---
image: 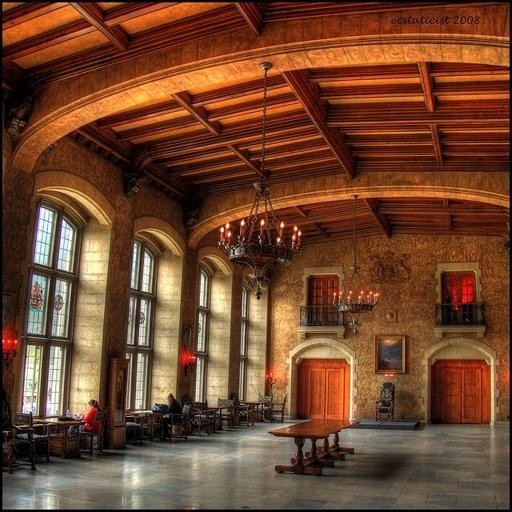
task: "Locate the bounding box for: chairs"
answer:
[1,392,287,474]
[375,381,394,421]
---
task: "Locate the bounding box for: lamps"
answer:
[264,371,278,387]
[0,325,21,365]
[184,350,198,376]
[330,191,379,335]
[214,60,306,300]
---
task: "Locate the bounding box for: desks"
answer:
[268,417,361,476]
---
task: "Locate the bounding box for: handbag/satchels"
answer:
[152,404,168,413]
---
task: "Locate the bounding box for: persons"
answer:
[66,398,102,439]
[158,393,183,438]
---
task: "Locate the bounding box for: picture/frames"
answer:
[375,335,405,374]
